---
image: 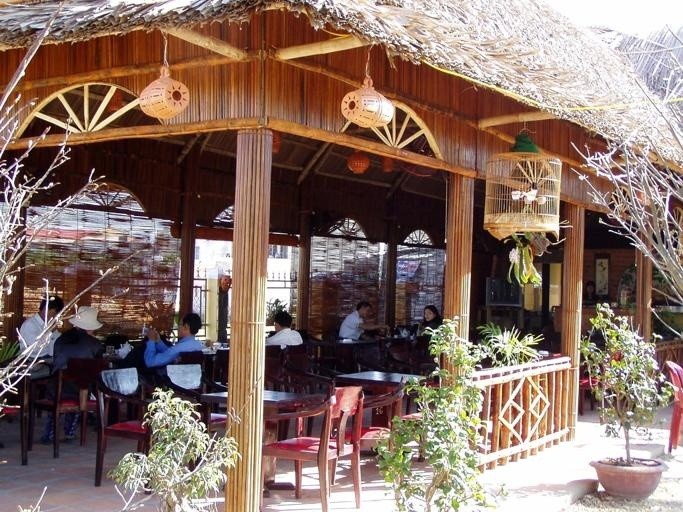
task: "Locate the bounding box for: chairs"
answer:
[665,360,683,453]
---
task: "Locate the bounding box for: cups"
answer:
[106,346,114,356]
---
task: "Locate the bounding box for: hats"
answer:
[68,306,103,331]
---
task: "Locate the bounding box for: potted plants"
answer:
[578,300,683,502]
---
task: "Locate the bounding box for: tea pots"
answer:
[120,341,133,351]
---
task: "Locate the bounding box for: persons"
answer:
[265,311,303,350]
[581,281,601,305]
[128,312,201,387]
[37,305,104,443]
[421,304,442,343]
[18,296,63,371]
[337,301,390,371]
